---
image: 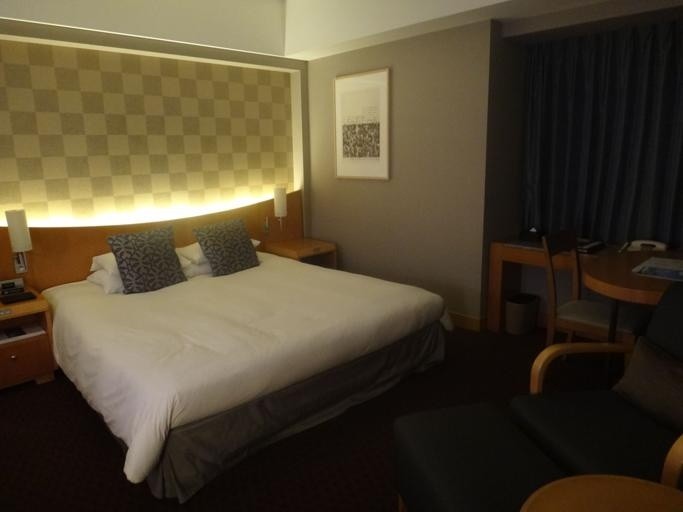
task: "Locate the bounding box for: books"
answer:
[632,257,683,281]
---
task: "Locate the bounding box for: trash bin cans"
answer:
[504,293,539,334]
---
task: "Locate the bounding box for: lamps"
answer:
[273,186,288,235]
[5,207,32,275]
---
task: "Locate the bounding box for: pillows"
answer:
[87,216,261,296]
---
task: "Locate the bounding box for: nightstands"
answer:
[262,239,338,270]
[0,286,58,391]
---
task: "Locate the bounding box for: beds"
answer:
[37,237,456,511]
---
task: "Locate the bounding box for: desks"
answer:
[486,236,602,334]
[581,248,683,341]
[519,473,683,512]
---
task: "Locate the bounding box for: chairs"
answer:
[542,236,650,377]
[384,282,683,510]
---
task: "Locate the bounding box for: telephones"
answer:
[627,239,667,252]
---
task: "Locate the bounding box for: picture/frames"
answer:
[331,66,391,181]
